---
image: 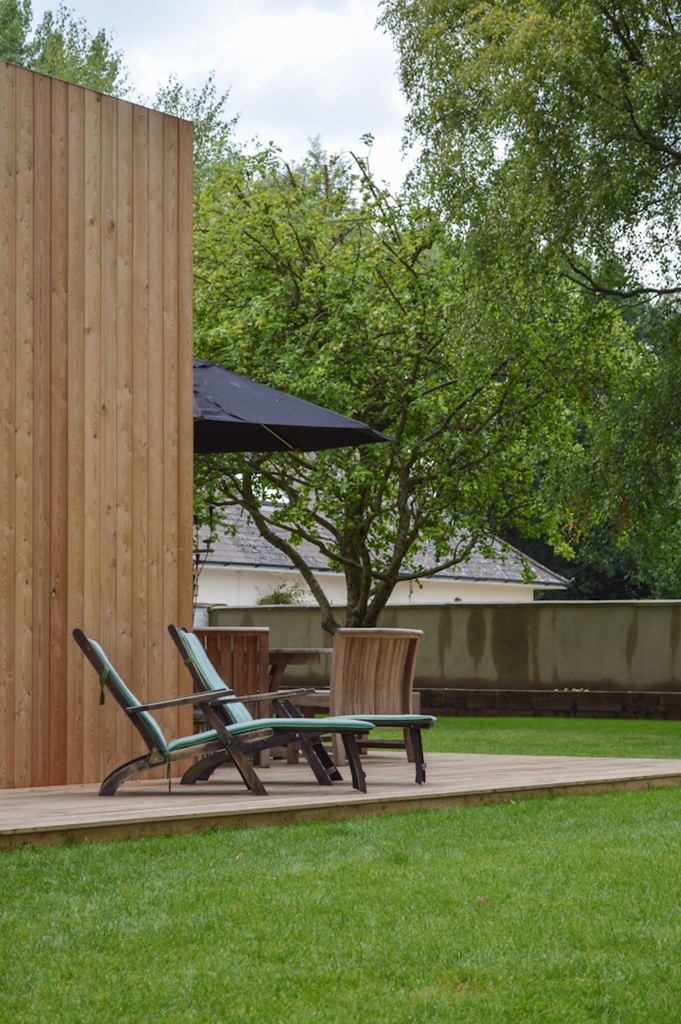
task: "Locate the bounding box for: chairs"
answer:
[72,627,375,793]
[193,627,272,767]
[168,623,437,785]
[286,627,424,766]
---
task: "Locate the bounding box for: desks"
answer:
[200,647,333,761]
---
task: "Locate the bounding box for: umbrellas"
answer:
[193,357,397,455]
